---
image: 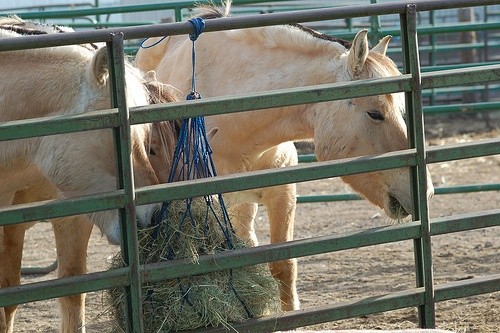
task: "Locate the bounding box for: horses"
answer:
[1,15,218,332]
[133,16,434,315]
